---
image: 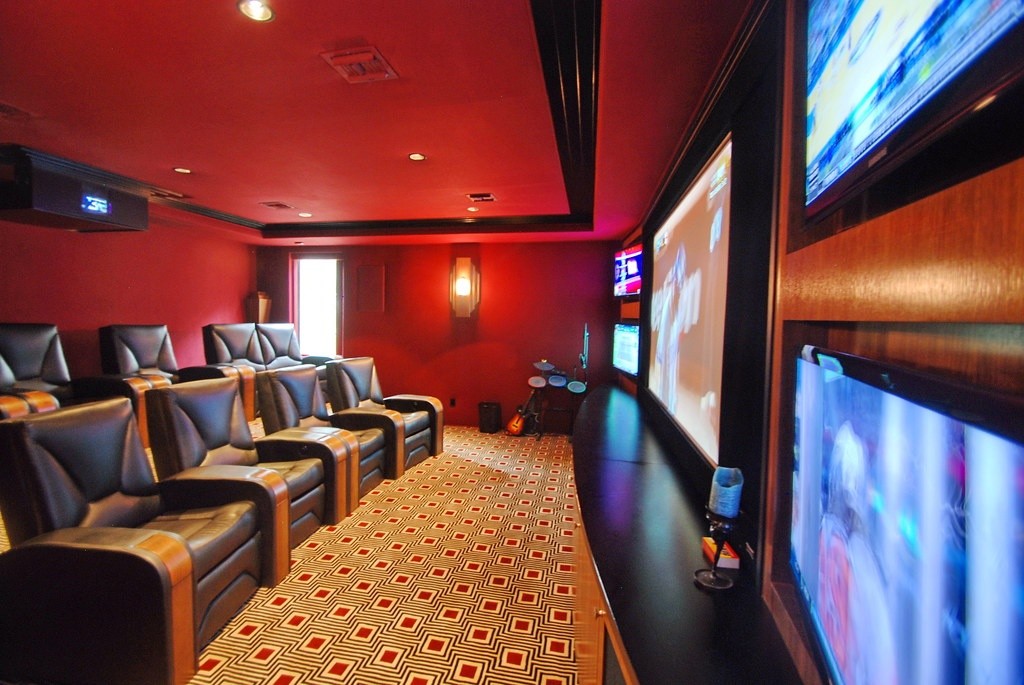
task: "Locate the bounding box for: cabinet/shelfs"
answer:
[569,378,800,685]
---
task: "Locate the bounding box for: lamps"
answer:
[448,256,480,319]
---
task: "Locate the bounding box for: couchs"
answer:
[0,320,444,685]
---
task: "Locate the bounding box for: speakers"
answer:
[478,402,501,433]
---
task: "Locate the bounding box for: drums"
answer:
[567,381,586,393]
[548,375,567,387]
[527,376,546,387]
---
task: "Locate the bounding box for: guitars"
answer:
[524,389,541,435]
[505,389,536,436]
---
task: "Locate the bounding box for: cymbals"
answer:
[533,362,555,371]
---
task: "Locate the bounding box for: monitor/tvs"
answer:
[611,113,761,501]
[788,345,1024,685]
[800,0,1024,228]
